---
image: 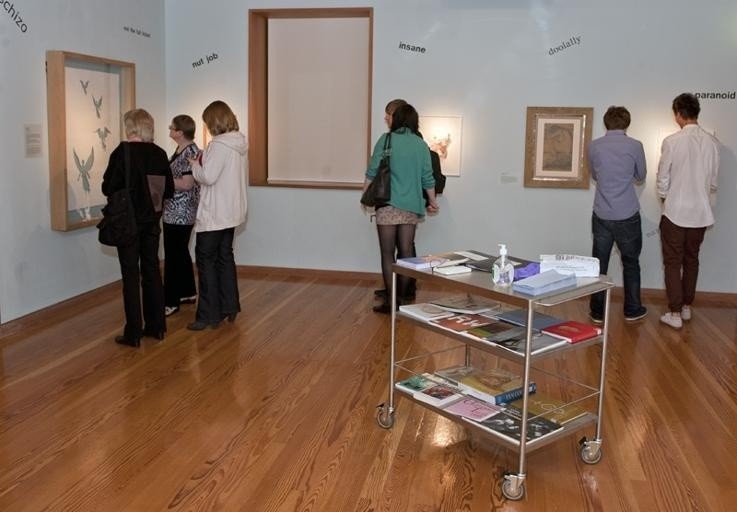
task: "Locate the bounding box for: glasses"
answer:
[169,126,175,130]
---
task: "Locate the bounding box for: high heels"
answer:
[219,311,237,322]
[187,320,219,330]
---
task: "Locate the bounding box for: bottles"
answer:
[491,243,514,288]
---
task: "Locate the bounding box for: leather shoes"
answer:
[375,290,386,296]
[142,328,164,340]
[373,303,399,313]
[115,336,141,347]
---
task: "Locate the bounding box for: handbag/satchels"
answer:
[96,188,137,246]
[361,133,391,206]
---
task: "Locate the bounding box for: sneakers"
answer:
[624,307,648,320]
[588,312,603,323]
[180,295,197,304]
[681,305,691,320]
[660,312,682,329]
[165,306,180,316]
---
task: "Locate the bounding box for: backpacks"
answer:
[422,147,445,208]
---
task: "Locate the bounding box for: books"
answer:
[391,364,588,445]
[399,292,602,356]
[395,249,577,296]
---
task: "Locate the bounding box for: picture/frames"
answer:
[418,113,464,178]
[524,106,593,189]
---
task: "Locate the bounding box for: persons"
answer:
[101,108,176,348]
[586,104,650,325]
[656,92,722,329]
[362,103,441,315]
[187,99,249,332]
[384,99,447,302]
[162,114,203,318]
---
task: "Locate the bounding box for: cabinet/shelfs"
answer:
[375,249,617,501]
